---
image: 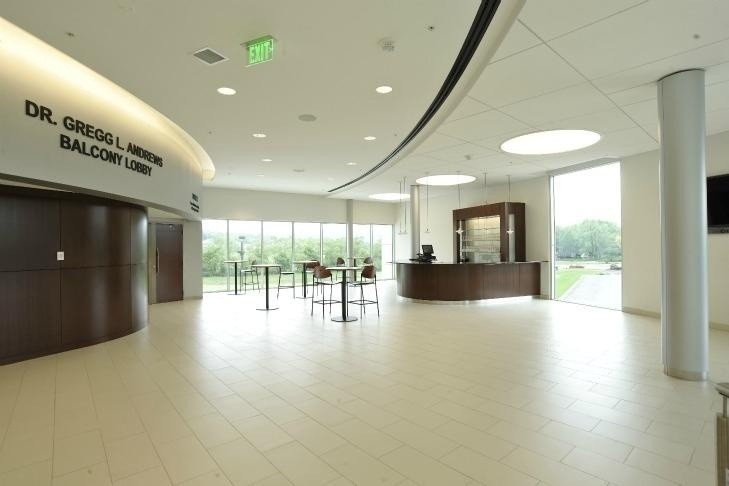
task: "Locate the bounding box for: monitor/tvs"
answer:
[707,174,729,234]
[422,245,433,255]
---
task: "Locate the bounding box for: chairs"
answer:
[224,258,381,324]
